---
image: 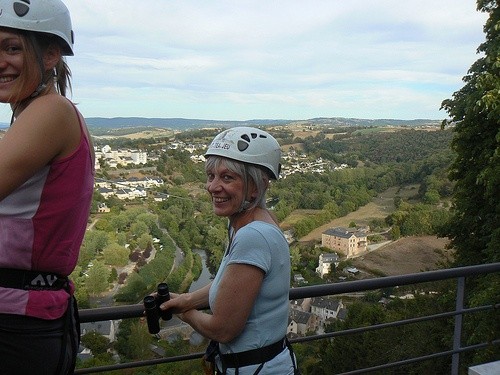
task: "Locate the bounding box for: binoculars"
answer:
[143,283,172,334]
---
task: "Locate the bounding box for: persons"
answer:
[0,0,94,375]
[146,127,298,375]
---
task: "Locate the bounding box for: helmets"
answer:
[0,0,74,56]
[203,127,281,181]
